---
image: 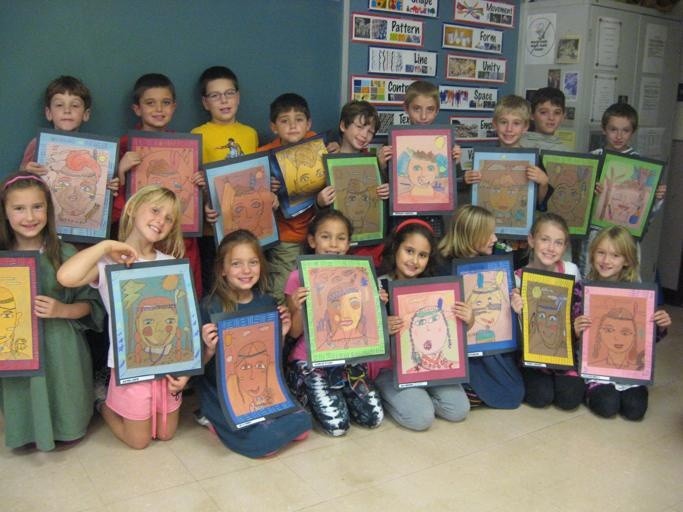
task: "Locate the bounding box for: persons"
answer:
[0,67,671,460]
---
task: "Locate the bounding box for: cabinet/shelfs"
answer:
[514,0,683,283]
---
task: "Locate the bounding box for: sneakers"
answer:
[191,407,208,428]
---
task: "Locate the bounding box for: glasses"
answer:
[350,121,374,138]
[203,89,238,99]
